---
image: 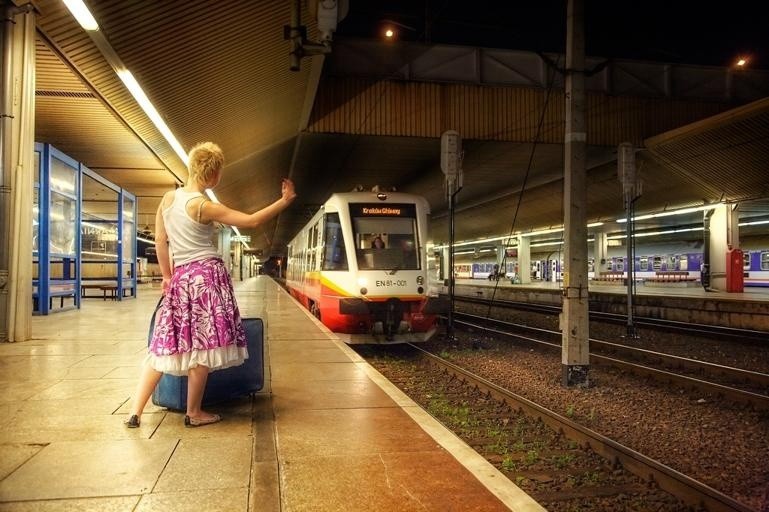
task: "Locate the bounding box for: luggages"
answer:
[148,317,265,411]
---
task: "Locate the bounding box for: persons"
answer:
[533,264,537,277]
[374,237,385,250]
[514,263,518,277]
[123,143,296,428]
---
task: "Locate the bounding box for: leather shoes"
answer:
[128,415,140,428]
[185,413,223,428]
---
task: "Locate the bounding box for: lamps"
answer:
[141,214,152,235]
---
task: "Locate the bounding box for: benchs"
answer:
[33,285,76,308]
[656,271,688,282]
[81,280,132,300]
[600,271,624,282]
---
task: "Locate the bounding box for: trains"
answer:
[435,249,769,287]
[284,183,441,347]
